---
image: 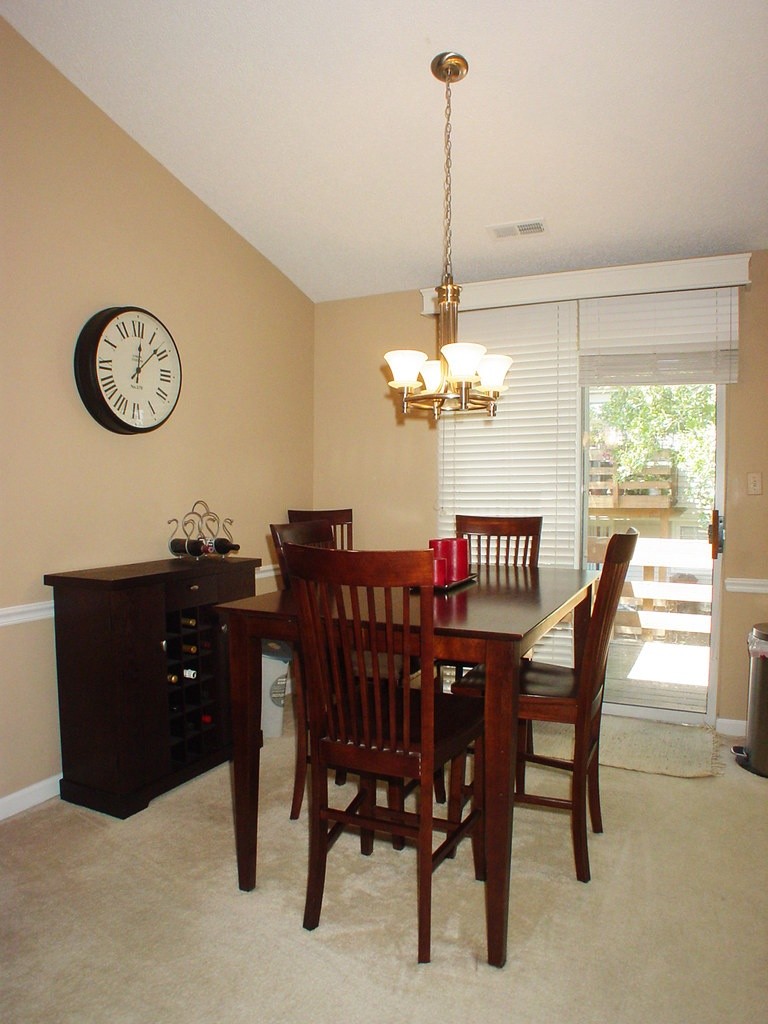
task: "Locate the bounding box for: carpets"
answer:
[530,714,725,777]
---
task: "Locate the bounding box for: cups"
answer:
[432,558,448,587]
[429,540,450,583]
[448,538,470,581]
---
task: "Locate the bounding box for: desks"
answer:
[213,565,605,968]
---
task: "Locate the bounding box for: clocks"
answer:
[73,306,182,435]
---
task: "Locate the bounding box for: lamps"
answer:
[383,51,514,421]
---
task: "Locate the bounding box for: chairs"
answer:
[266,505,435,822]
[455,512,546,755]
[278,539,484,964]
[448,526,640,883]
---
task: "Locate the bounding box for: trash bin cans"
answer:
[729,622,768,778]
[261,640,291,736]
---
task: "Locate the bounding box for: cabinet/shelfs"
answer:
[41,557,266,821]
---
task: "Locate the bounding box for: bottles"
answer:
[198,537,240,555]
[170,538,214,559]
